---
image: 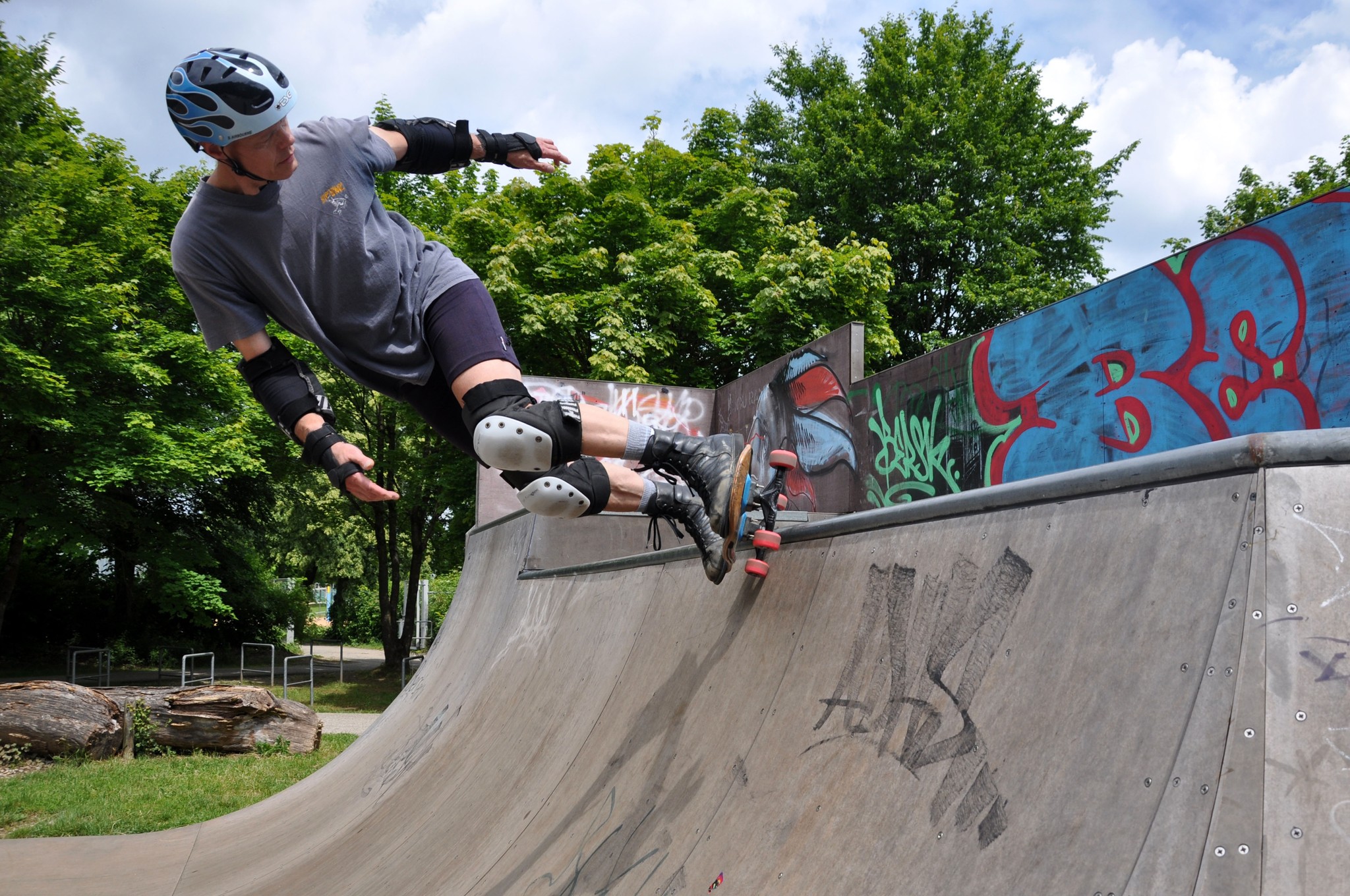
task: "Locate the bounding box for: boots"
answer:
[642,479,728,586]
[639,426,744,538]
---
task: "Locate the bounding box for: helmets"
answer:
[166,47,296,154]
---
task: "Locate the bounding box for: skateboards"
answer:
[724,445,796,577]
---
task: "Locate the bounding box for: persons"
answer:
[129,45,755,591]
